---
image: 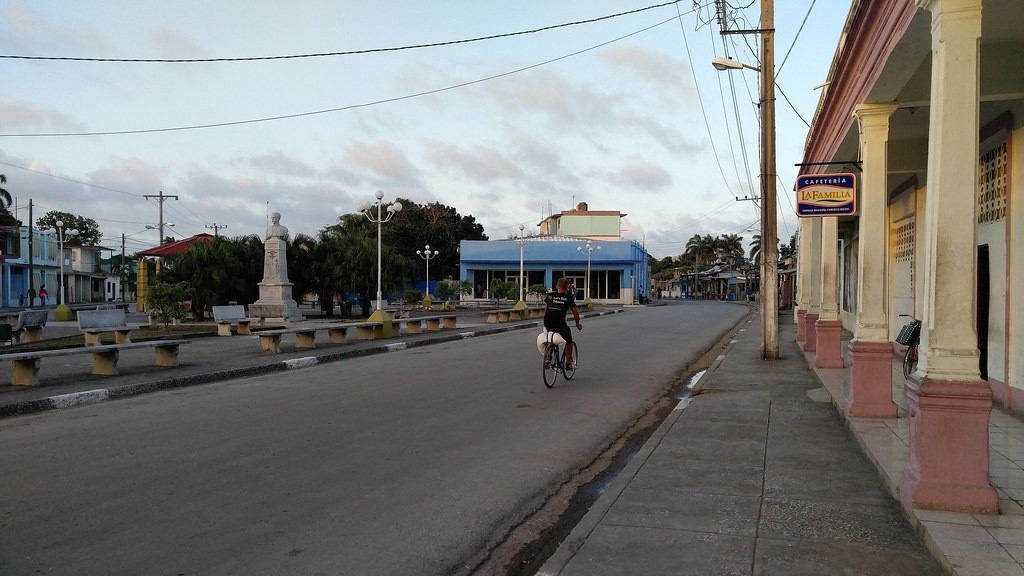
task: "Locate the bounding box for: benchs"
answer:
[249,303,292,329]
[0,339,193,387]
[482,306,544,323]
[69,306,98,320]
[77,309,140,347]
[10,309,48,341]
[115,304,130,312]
[571,302,589,313]
[96,305,116,310]
[257,321,384,355]
[392,315,457,337]
[212,305,252,336]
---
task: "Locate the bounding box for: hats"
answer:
[640,285,642,287]
[556,278,568,290]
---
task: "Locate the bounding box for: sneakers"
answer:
[544,360,551,371]
[566,362,573,370]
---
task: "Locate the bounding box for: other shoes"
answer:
[747,302,749,303]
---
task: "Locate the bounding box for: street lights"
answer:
[576,240,602,298]
[43,221,79,305]
[416,244,440,297]
[357,190,402,310]
[506,223,536,301]
[144,221,177,317]
[711,56,782,360]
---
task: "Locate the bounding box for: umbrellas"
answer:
[137,233,214,256]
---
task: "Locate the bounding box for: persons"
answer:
[544,278,583,371]
[746,286,751,303]
[726,289,734,301]
[266,212,288,237]
[39,284,48,306]
[639,285,653,304]
[658,287,662,298]
[26,286,37,308]
[19,294,25,309]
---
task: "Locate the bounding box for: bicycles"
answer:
[541,325,583,389]
[898,313,922,380]
